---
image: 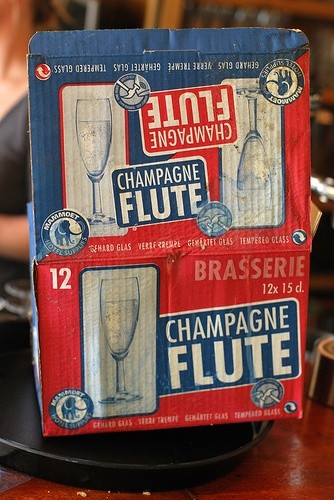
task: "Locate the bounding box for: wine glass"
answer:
[236,85,277,187]
[97,273,149,407]
[70,95,118,228]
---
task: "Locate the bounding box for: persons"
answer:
[303,129,333,307]
[0,0,84,289]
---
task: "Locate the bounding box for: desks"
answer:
[1,360,333,499]
